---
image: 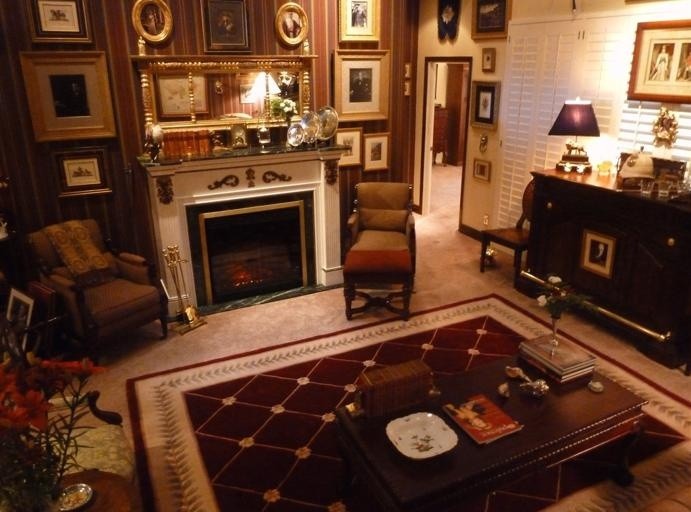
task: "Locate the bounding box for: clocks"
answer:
[436,0,462,44]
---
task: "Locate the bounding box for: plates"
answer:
[317,106,338,140]
[286,124,305,147]
[387,412,458,461]
[57,484,93,511]
[301,111,320,143]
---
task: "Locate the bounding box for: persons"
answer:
[16,304,26,327]
[218,11,236,34]
[590,241,607,265]
[648,43,675,82]
[283,11,301,38]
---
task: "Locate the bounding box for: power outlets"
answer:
[483,214,488,225]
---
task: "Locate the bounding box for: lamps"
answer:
[547,96,600,173]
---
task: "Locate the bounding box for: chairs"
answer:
[479,179,533,282]
[432,106,456,165]
[342,182,415,321]
[22,214,167,365]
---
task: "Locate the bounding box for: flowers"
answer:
[1,357,122,511]
[279,96,298,124]
[538,275,588,319]
[267,96,282,119]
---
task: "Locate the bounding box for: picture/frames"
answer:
[362,132,391,174]
[155,74,210,118]
[333,127,362,168]
[239,82,255,104]
[338,0,380,43]
[626,19,691,103]
[199,0,254,54]
[17,50,116,142]
[274,1,308,49]
[482,48,497,72]
[470,0,510,39]
[473,158,492,182]
[131,0,173,43]
[333,48,393,123]
[470,80,500,131]
[578,228,617,279]
[0,283,37,361]
[25,0,94,44]
[47,145,115,202]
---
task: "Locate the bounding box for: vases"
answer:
[283,119,293,147]
[551,317,562,346]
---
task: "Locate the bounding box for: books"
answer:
[441,392,524,447]
[518,332,597,384]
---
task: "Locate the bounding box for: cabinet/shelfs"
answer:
[513,168,690,369]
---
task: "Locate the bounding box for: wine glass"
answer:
[258,128,271,153]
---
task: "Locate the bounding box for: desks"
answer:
[1,469,140,512]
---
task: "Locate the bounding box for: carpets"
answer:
[125,292,691,512]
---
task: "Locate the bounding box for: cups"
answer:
[658,180,673,196]
[641,179,654,196]
